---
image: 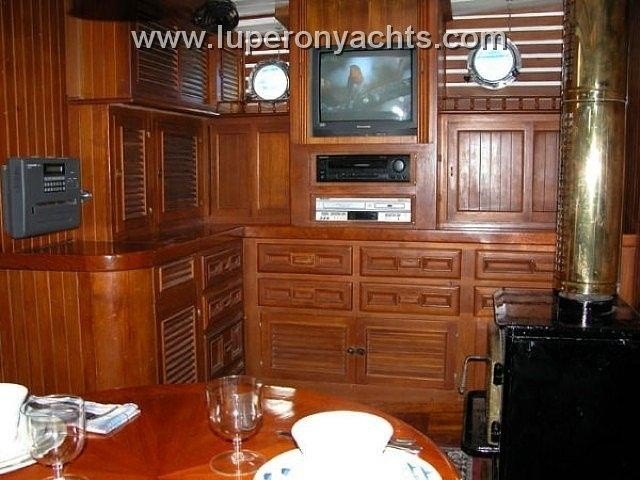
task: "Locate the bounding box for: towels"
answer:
[26,393,144,435]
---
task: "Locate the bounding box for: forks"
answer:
[85,406,118,420]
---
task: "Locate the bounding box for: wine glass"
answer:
[19,393,89,480]
[203,375,270,477]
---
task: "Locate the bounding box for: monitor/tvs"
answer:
[312,42,417,137]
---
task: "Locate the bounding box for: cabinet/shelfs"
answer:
[70,105,202,242]
[64,3,224,108]
[201,241,251,381]
[474,251,558,386]
[221,45,241,113]
[0,254,202,388]
[446,119,557,223]
[208,121,293,226]
[255,242,462,396]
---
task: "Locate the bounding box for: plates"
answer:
[0,412,65,475]
[253,447,442,480]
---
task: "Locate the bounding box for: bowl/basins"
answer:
[290,410,396,468]
[0,383,29,447]
